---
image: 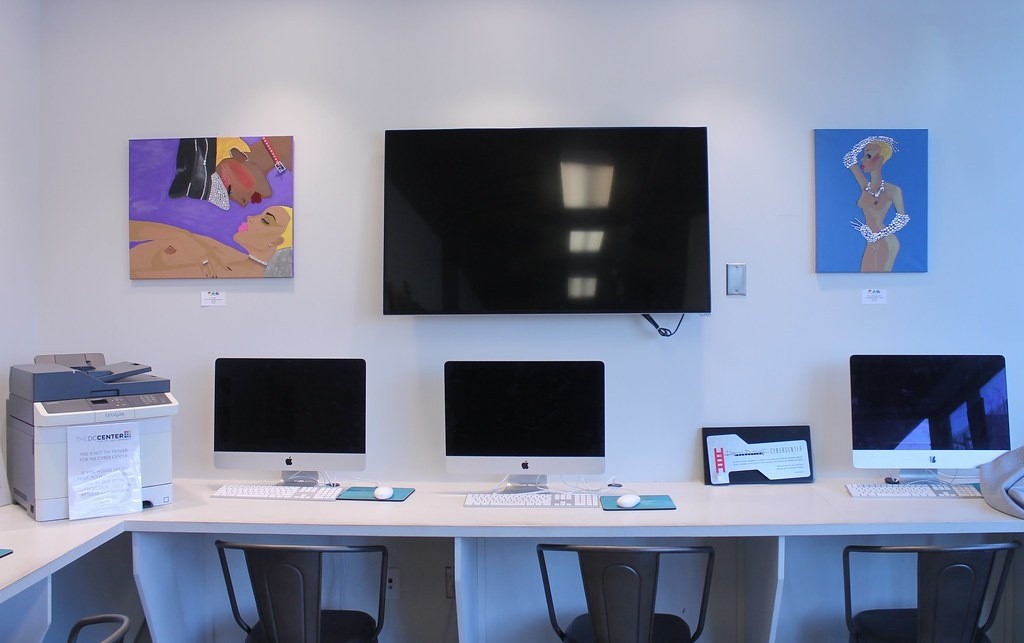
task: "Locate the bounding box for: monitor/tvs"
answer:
[382,126,713,315]
[214,357,366,487]
[848,354,1011,483]
[443,360,605,493]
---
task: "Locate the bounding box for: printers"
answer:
[6,353,179,522]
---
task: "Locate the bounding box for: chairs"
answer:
[535,544,716,643]
[215,540,388,643]
[843,541,1021,643]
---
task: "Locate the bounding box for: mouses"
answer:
[617,494,641,508]
[374,486,393,499]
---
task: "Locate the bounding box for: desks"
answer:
[0,484,1024,643]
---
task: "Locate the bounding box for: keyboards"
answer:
[464,494,600,508]
[845,484,982,498]
[210,484,343,503]
